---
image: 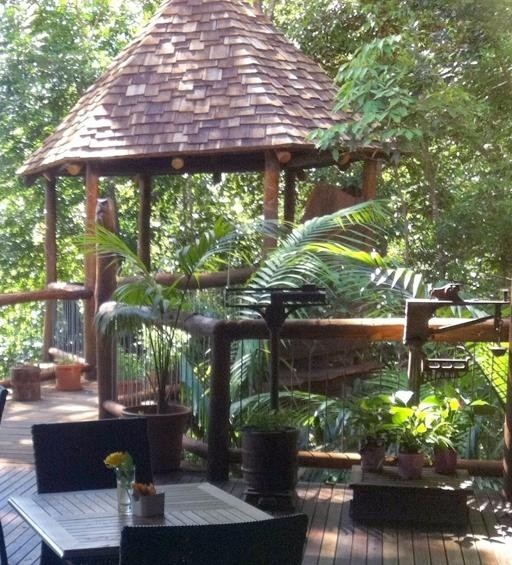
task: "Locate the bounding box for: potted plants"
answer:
[240,407,301,493]
[0,342,86,406]
[73,215,249,471]
[336,389,500,480]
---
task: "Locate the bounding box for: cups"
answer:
[130,489,165,517]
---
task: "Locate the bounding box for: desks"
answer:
[346,465,471,526]
